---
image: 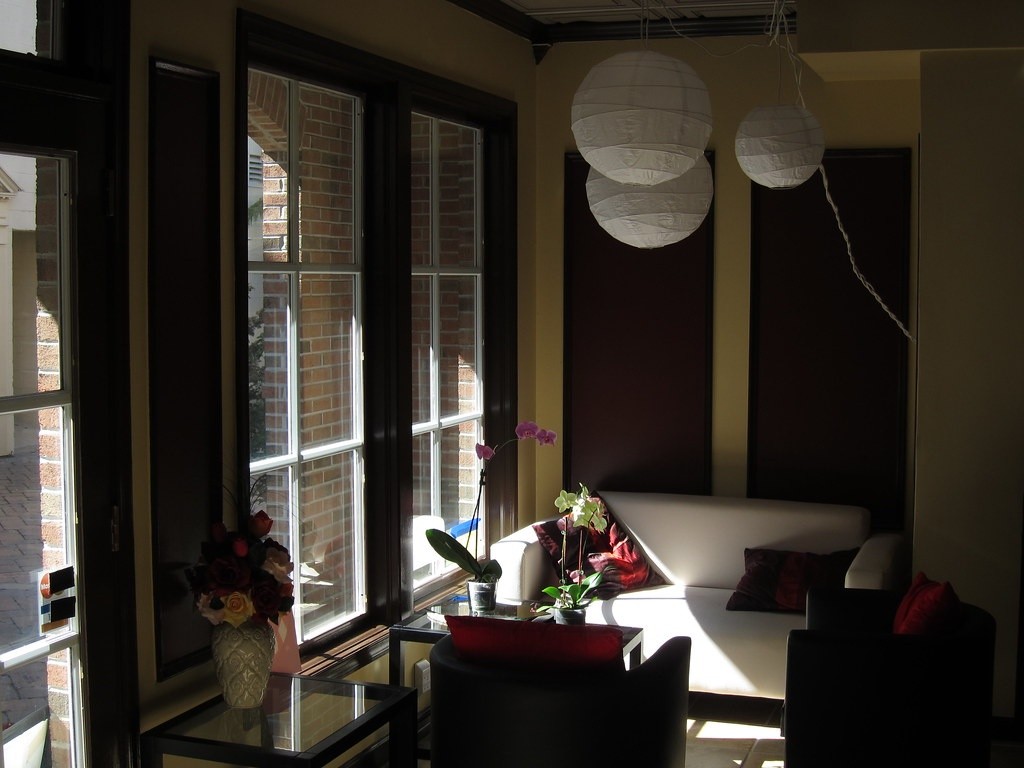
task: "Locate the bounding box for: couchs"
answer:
[491,491,904,701]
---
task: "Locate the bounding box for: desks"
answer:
[390,598,645,768]
[141,671,420,768]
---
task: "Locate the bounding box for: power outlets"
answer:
[414,658,430,695]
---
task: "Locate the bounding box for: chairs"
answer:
[783,583,997,768]
[429,634,690,768]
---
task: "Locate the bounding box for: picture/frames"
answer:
[747,147,911,535]
[562,148,715,495]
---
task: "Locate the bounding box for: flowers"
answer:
[188,471,294,628]
[536,483,608,618]
[427,420,557,583]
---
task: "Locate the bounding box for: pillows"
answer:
[444,615,626,675]
[725,547,860,613]
[893,570,957,635]
[537,492,665,601]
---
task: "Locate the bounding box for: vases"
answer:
[468,580,496,610]
[210,624,275,708]
[554,606,586,626]
